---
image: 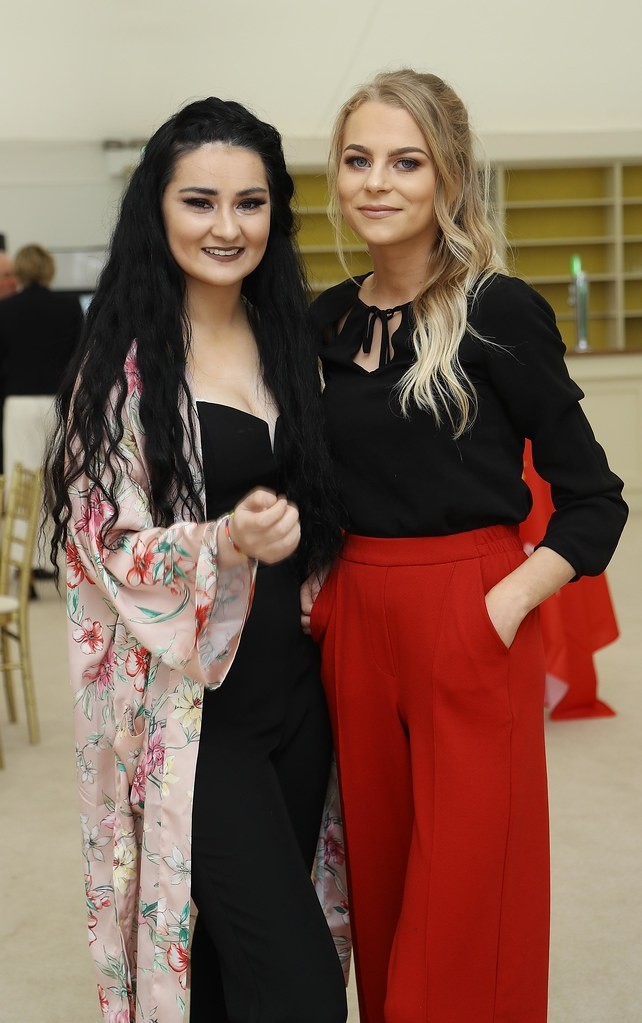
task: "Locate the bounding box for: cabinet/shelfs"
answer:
[283,154,642,351]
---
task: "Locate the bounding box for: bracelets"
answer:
[225,512,240,553]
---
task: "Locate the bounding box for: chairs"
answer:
[0,463,43,746]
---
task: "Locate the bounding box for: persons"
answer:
[36,69,629,1022]
[0,234,86,601]
